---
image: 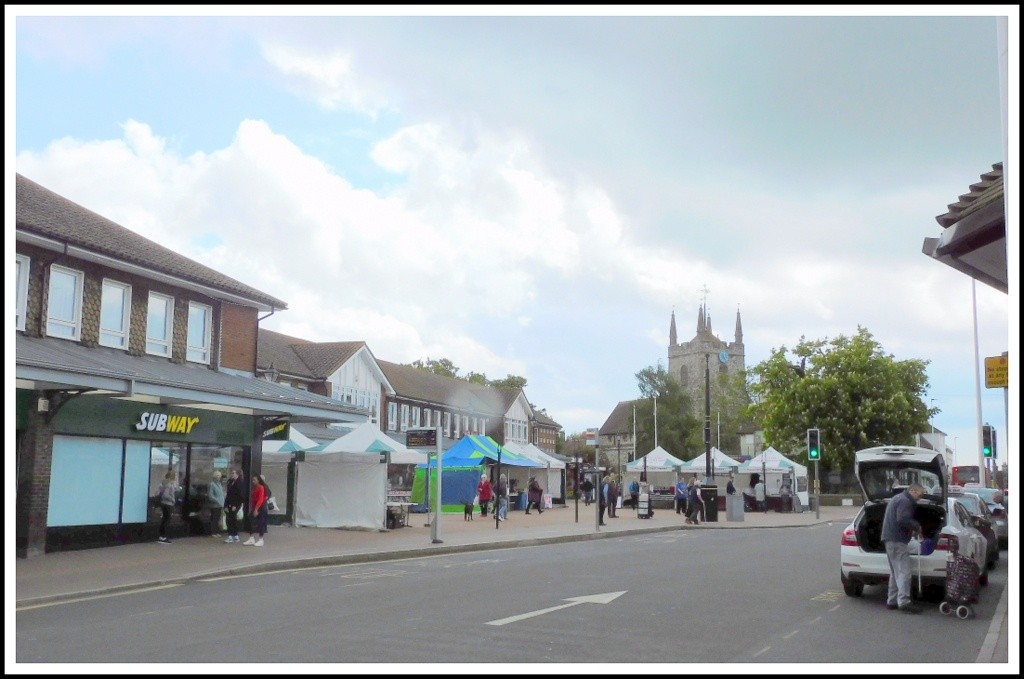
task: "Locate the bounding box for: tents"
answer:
[621,446,810,510]
[263,422,428,532]
[411,434,568,514]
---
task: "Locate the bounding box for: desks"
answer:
[387,502,418,527]
[650,495,677,509]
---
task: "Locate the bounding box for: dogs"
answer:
[464,503,474,520]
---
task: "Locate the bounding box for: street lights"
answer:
[931,399,935,449]
[652,389,660,448]
[496,442,502,529]
[573,438,579,522]
[953,437,958,466]
[617,435,623,508]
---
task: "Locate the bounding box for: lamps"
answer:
[265,362,279,382]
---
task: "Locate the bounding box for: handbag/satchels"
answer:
[266,496,280,513]
[267,502,274,510]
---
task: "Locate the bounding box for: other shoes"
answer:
[243,535,256,545]
[224,535,241,544]
[898,602,924,614]
[886,603,898,610]
[255,537,264,546]
[157,536,171,544]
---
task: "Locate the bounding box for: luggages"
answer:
[938,546,979,619]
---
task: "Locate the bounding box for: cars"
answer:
[892,483,1008,571]
[841,445,988,597]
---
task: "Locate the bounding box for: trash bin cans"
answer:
[700,484,718,522]
[725,494,745,522]
[517,488,528,511]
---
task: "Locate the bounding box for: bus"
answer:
[953,466,991,488]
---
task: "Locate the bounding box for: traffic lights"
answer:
[983,426,997,459]
[807,429,821,460]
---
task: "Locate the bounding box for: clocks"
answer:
[719,351,728,362]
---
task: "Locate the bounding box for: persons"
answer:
[881,484,922,614]
[477,474,507,521]
[190,484,198,504]
[993,493,1008,514]
[582,478,594,506]
[224,467,245,543]
[676,476,704,524]
[243,475,272,546]
[755,480,764,513]
[599,475,618,526]
[726,477,736,495]
[525,476,544,514]
[629,477,639,511]
[208,471,227,537]
[159,471,181,542]
